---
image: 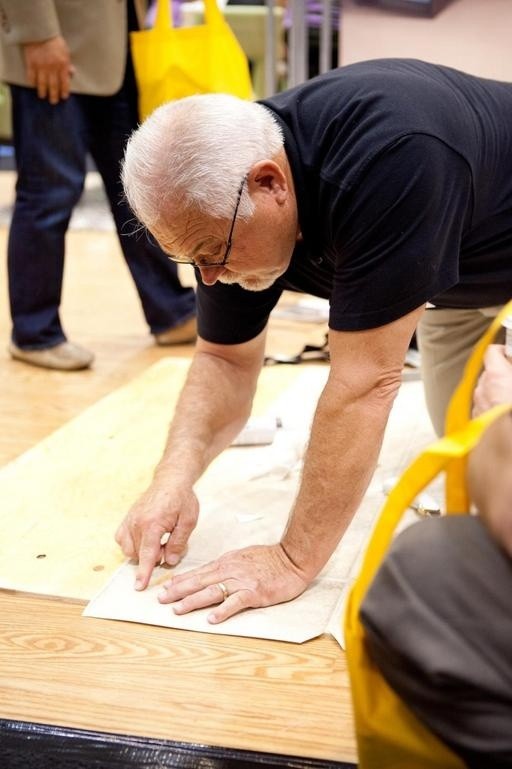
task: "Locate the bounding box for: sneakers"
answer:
[157,316,197,344]
[10,341,91,371]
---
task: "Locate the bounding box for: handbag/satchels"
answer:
[130,0,254,123]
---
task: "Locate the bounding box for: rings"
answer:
[217,583,228,601]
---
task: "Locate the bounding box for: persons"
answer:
[0,0,197,371]
[359,343,512,769]
[117,60,512,626]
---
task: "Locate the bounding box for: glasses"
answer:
[168,181,244,266]
